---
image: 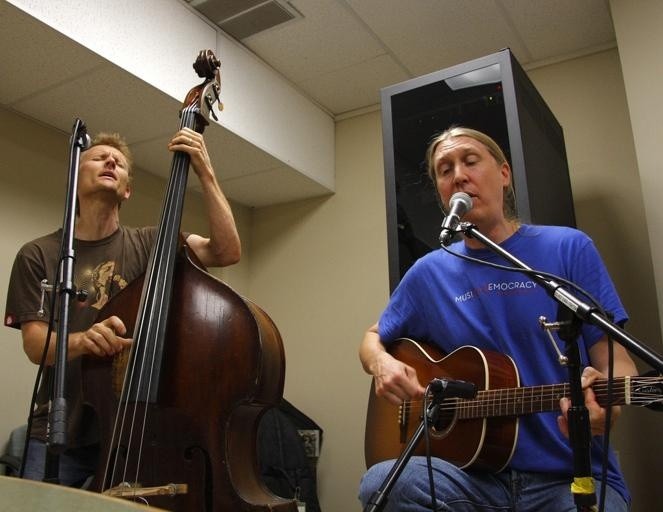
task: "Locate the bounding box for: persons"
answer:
[4,126,241,487]
[358,127,639,512]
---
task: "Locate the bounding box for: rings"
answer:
[189,138,192,145]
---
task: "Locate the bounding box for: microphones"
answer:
[439,190,474,244]
[69,117,93,150]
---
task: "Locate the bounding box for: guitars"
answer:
[364,338,662,472]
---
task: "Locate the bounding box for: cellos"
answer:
[81,48,300,512]
